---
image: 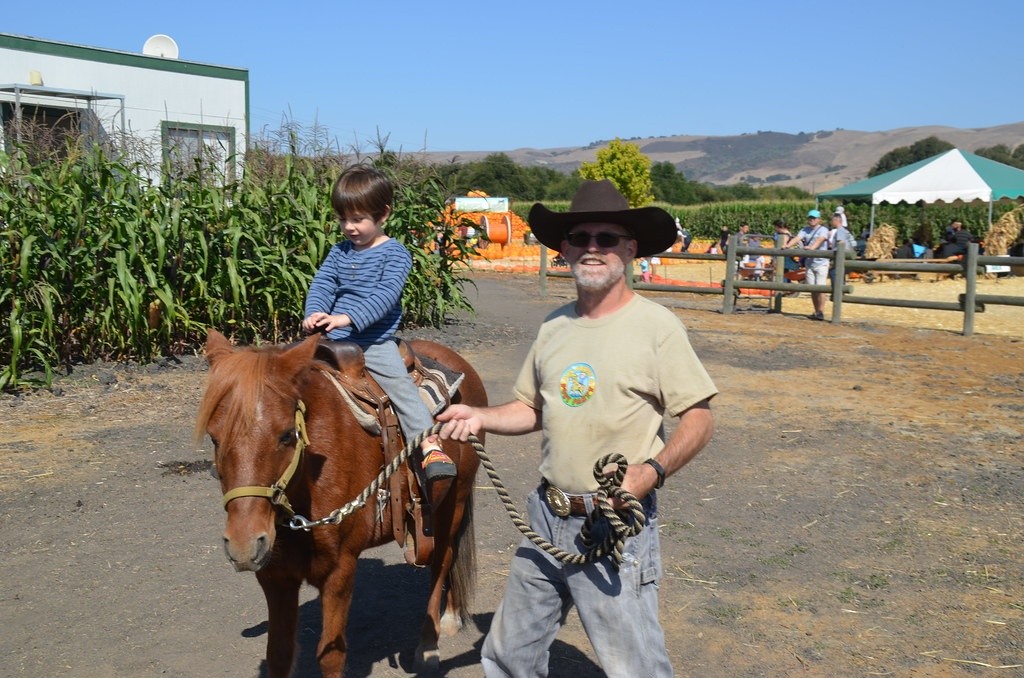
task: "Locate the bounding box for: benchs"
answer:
[874,258,953,283]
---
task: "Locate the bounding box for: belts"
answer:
[541,477,599,516]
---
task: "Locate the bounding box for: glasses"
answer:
[565,230,635,248]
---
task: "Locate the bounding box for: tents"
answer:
[815,148,1024,239]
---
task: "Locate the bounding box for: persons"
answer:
[861,225,870,240]
[410,218,476,266]
[827,206,853,301]
[893,220,985,259]
[428,180,719,678]
[720,219,801,296]
[781,210,830,320]
[302,164,456,483]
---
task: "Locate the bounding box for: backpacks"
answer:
[780,233,800,263]
[679,230,690,246]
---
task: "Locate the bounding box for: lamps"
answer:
[32,71,41,86]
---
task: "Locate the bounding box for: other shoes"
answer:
[808,313,823,321]
[420,449,457,482]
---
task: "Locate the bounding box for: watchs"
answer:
[644,459,666,489]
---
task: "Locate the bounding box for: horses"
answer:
[203,327,488,678]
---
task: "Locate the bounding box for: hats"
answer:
[528,178,677,259]
[808,210,820,218]
